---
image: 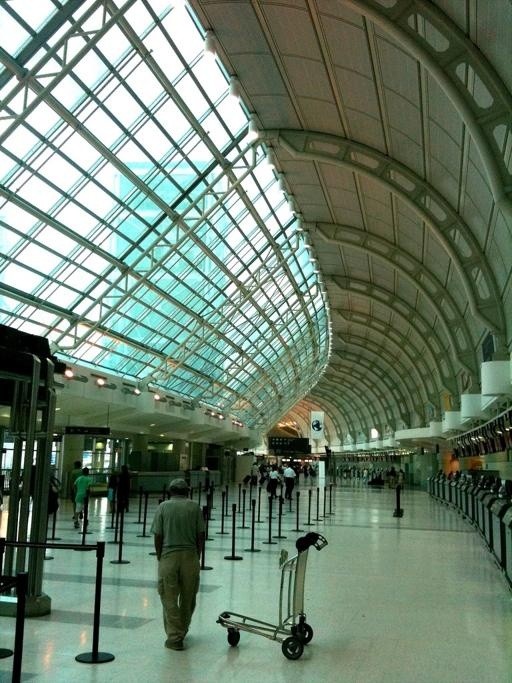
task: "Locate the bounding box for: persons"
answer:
[248,458,405,499]
[45,460,130,525]
[150,476,206,649]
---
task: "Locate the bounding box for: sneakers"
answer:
[165,639,184,650]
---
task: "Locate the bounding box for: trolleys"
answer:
[216,531,327,660]
